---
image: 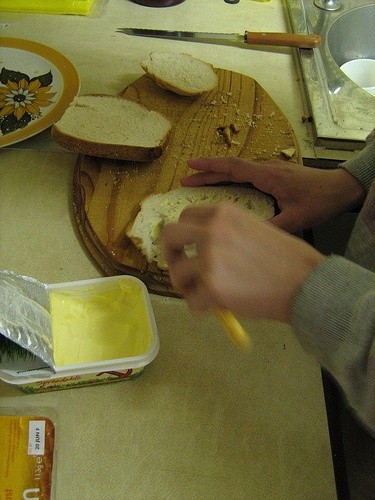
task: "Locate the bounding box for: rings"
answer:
[183,243,199,259]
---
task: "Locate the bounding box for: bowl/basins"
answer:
[339,59,374,95]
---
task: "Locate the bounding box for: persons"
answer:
[159,130,375,436]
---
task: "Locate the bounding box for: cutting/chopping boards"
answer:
[74,69,303,298]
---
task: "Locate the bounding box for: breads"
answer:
[51,93,174,160]
[125,182,278,270]
[141,51,219,95]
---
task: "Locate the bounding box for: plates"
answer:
[0,36,81,149]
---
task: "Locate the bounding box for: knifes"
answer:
[117,27,321,48]
